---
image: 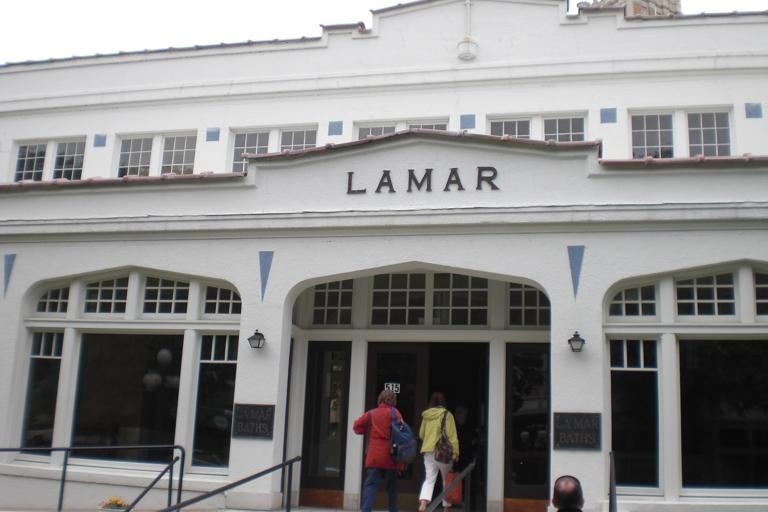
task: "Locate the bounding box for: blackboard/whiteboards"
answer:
[232,404,275,440]
[554,412,601,450]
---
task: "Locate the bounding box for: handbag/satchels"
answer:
[435,438,453,464]
[389,422,417,464]
[446,473,463,505]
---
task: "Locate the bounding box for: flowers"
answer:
[96,495,134,509]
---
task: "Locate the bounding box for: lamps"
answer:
[568,331,585,352]
[246,328,265,349]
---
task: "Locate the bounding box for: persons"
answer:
[417,391,459,512]
[552,476,584,512]
[353,390,403,512]
[455,404,481,473]
